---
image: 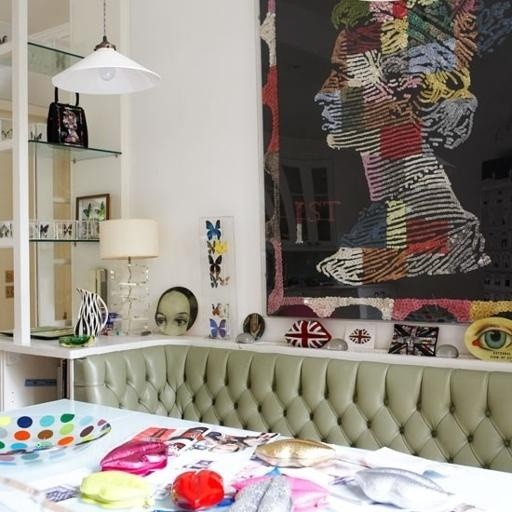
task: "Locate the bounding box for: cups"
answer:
[0,217,100,240]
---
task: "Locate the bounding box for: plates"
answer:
[0,404,113,468]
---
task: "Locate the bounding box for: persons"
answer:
[154,285,198,338]
[313,1,511,286]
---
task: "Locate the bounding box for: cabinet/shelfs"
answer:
[1,1,123,408]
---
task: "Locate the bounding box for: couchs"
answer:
[75,345,512,473]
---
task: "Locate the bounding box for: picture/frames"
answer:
[74,193,109,221]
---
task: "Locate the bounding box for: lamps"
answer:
[51,0,161,97]
[98,218,159,340]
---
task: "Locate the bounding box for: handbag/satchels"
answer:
[46,86,89,150]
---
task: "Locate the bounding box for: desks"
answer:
[1,399,512,512]
